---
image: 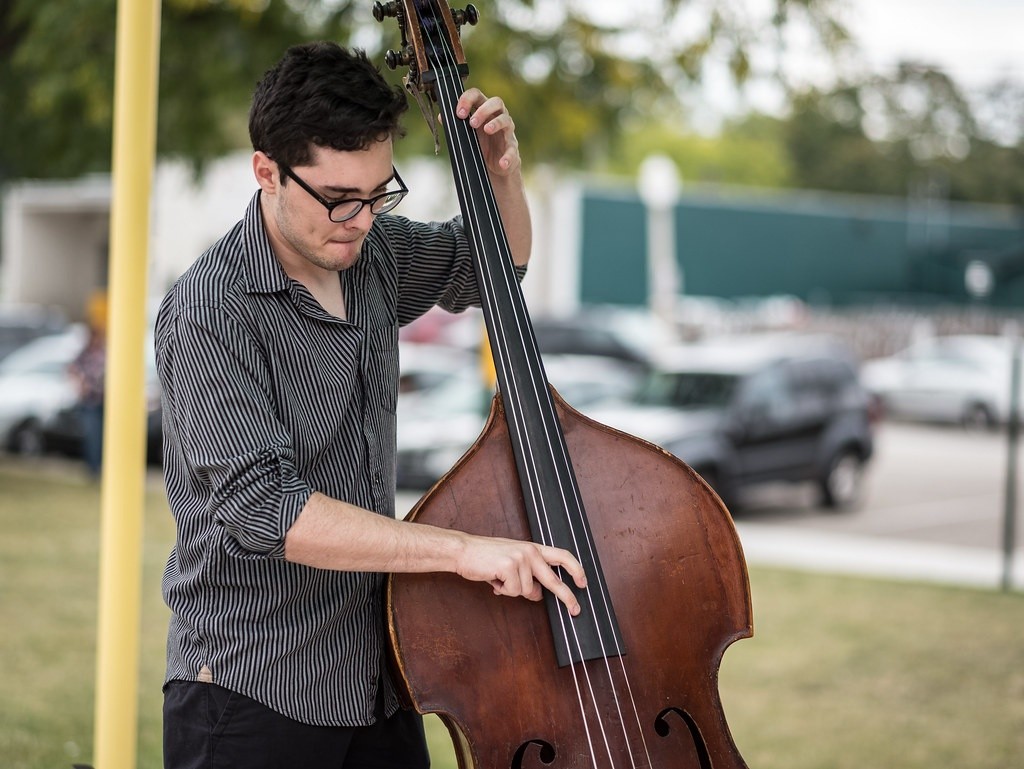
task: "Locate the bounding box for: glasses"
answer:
[279,161,410,224]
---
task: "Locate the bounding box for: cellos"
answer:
[370,1,758,769]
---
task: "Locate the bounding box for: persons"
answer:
[66,296,104,476]
[154,40,587,769]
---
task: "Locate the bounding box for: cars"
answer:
[857,332,1023,432]
[2,311,876,511]
